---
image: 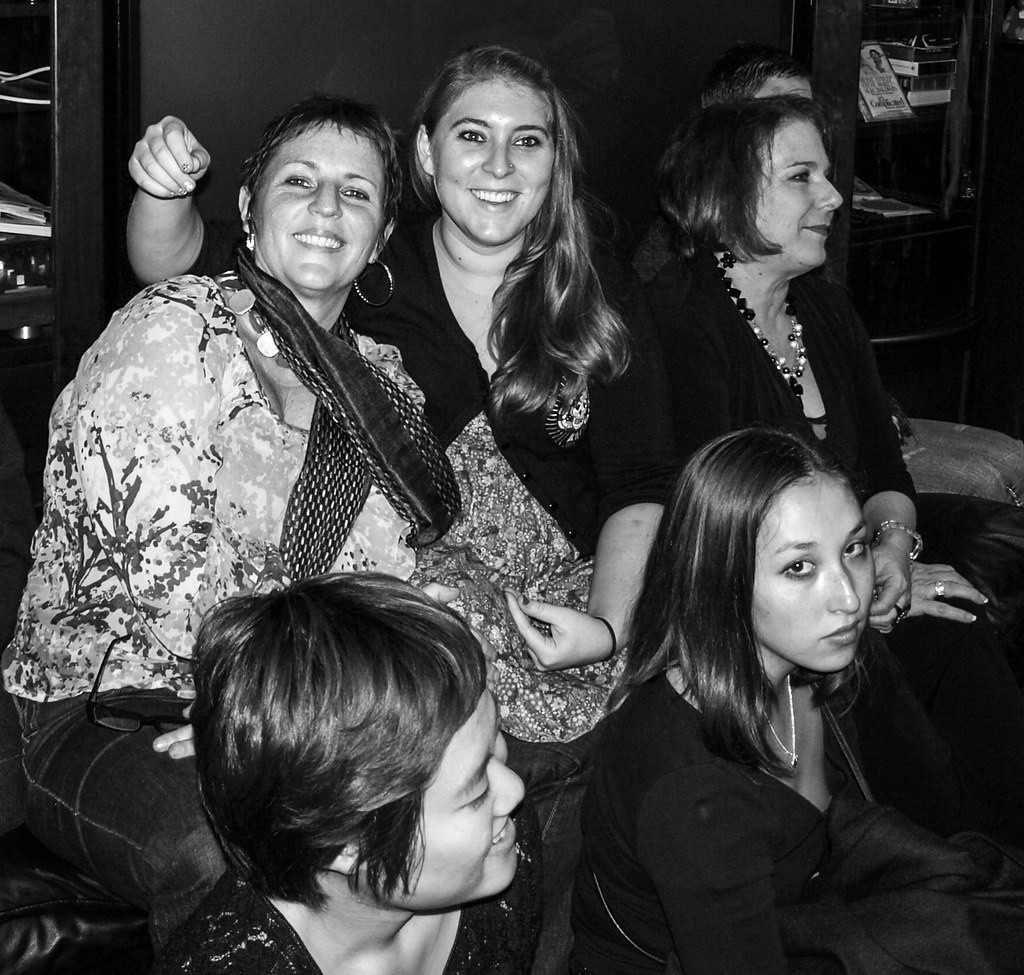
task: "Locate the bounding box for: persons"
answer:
[123,45,669,672]
[587,94,1024,851]
[629,40,1022,505]
[566,426,1024,975]
[160,573,546,975]
[870,48,887,74]
[0,95,500,958]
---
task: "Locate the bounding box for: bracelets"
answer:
[869,520,923,564]
[590,616,616,662]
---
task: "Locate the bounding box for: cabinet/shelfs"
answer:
[0,0,61,411]
[782,0,1005,425]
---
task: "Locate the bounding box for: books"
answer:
[0,63,53,112]
[880,39,958,108]
[0,179,51,296]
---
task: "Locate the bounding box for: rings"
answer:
[893,602,911,625]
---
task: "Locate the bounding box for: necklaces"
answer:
[717,259,807,396]
